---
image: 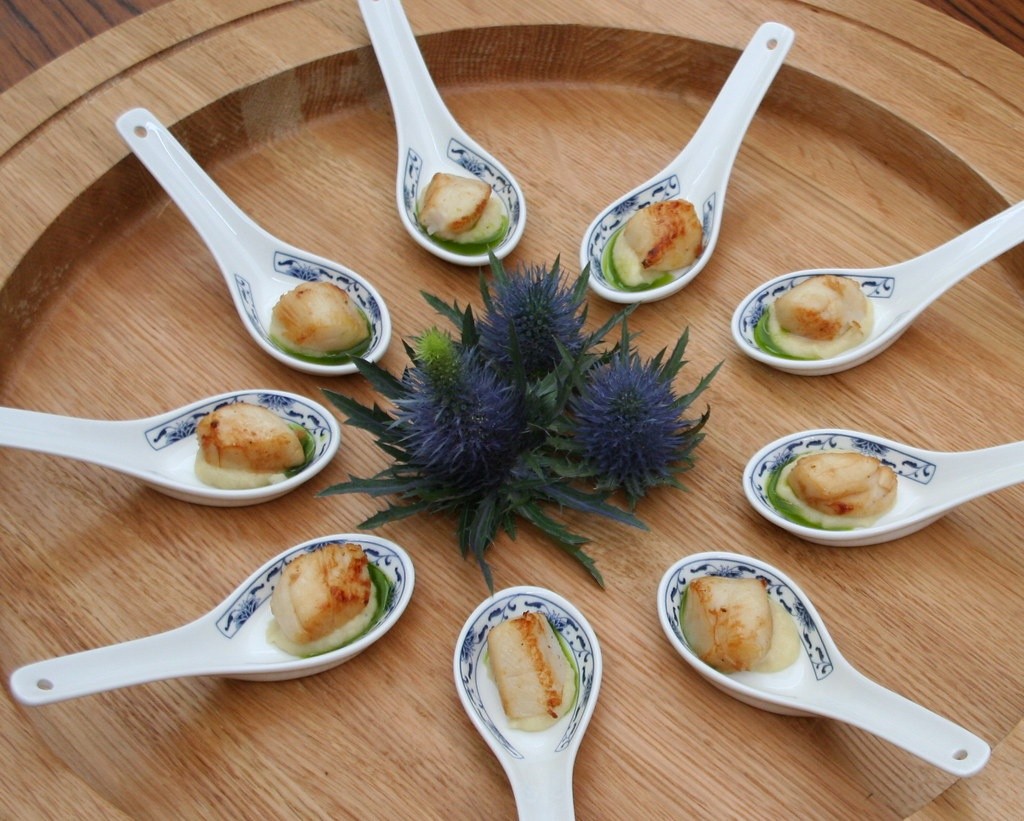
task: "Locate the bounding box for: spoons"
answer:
[10,532,414,706]
[656,551,991,778]
[115,106,392,376]
[578,21,795,304]
[358,0,527,264]
[743,428,1024,546]
[732,200,1023,376]
[0,389,341,507]
[452,585,602,821]
